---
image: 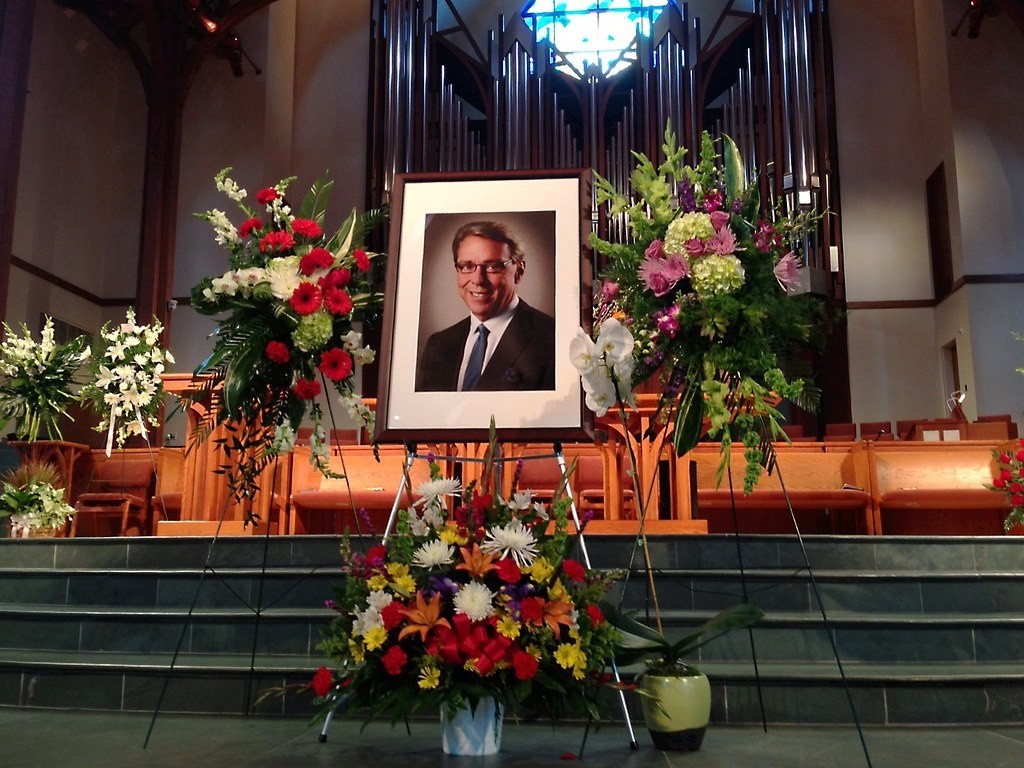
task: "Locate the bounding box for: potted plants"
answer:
[599,596,766,754]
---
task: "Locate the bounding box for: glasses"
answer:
[454,258,513,273]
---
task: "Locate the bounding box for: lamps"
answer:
[950,0,1003,40]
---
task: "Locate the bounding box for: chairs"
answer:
[859,421,894,441]
[294,428,325,445]
[896,418,928,441]
[764,424,818,442]
[70,460,156,537]
[329,429,359,445]
[823,423,857,442]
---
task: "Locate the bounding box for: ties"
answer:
[462,324,490,391]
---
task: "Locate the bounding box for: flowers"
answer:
[164,165,390,531]
[568,116,838,498]
[79,306,176,449]
[980,438,1024,535]
[249,414,671,745]
[0,480,79,538]
[0,313,91,445]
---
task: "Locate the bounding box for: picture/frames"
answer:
[373,168,597,444]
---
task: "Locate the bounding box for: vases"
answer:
[439,697,504,756]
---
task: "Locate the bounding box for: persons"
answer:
[414,220,555,391]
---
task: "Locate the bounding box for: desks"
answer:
[159,374,279,536]
[595,393,782,520]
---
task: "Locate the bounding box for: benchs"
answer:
[503,443,643,520]
[867,440,1021,536]
[288,445,447,534]
[672,440,874,535]
[150,447,292,535]
